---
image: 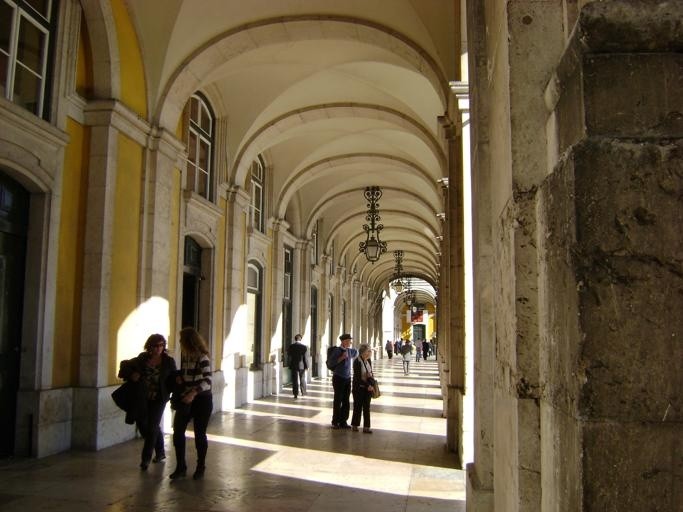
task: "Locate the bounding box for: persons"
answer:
[428,340,434,356]
[385,337,404,358]
[414,337,421,362]
[422,339,428,359]
[166,326,213,481]
[123,333,177,470]
[285,333,310,398]
[348,343,373,434]
[323,333,358,430]
[401,339,411,376]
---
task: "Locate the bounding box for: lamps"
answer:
[359,186,387,264]
[392,250,419,314]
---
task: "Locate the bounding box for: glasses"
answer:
[154,343,164,347]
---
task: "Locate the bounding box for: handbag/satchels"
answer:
[326,346,336,372]
[170,386,190,410]
[367,376,380,400]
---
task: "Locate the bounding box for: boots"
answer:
[193,466,205,480]
[169,465,187,479]
[363,427,372,434]
[332,422,359,432]
[140,455,166,471]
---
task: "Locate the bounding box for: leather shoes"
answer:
[294,392,307,398]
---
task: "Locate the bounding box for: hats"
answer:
[339,334,353,340]
